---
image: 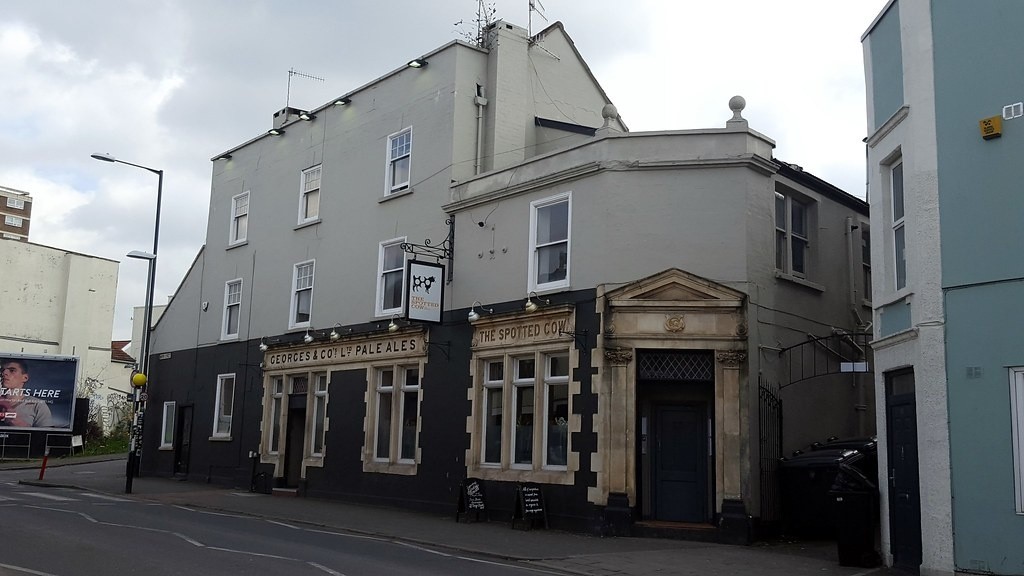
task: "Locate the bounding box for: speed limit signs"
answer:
[140,392,149,402]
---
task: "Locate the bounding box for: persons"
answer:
[0,359,55,426]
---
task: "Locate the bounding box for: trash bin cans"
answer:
[830,452,879,568]
[812,434,877,449]
[775,449,858,542]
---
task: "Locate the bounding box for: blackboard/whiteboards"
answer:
[511,482,546,520]
[456,477,489,513]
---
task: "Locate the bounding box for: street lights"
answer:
[91,152,163,477]
[124,250,157,494]
[125,374,147,492]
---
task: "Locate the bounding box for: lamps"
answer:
[389,314,411,333]
[467,300,493,323]
[260,336,280,353]
[299,112,317,121]
[330,323,353,342]
[268,128,286,136]
[526,291,550,314]
[334,97,351,106]
[218,154,232,160]
[304,327,326,343]
[408,59,428,68]
